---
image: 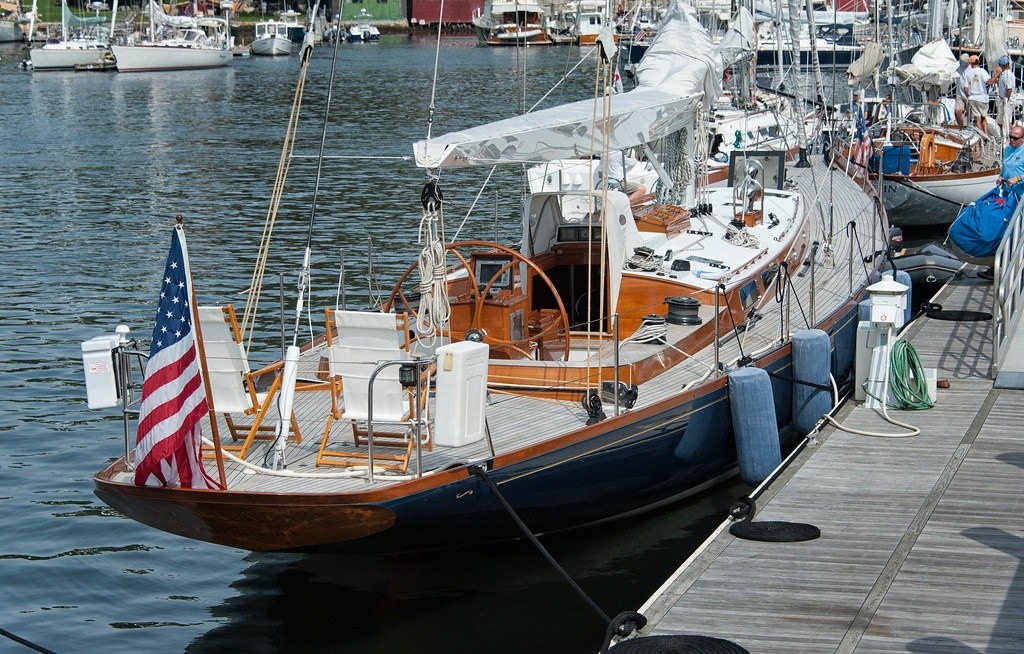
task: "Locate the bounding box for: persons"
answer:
[996,125,1024,187]
[841,90,892,138]
[954,52,969,131]
[996,55,1016,135]
[964,55,1002,146]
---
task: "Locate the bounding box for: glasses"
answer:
[1009,135,1023,141]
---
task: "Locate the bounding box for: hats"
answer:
[968,55,979,62]
[959,53,969,63]
[998,57,1009,65]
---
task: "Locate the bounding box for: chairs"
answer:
[199,303,437,472]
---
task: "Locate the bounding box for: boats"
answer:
[0,1,1024,554]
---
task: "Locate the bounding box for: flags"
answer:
[134,223,226,491]
[854,104,873,177]
[634,23,645,42]
[614,63,623,93]
